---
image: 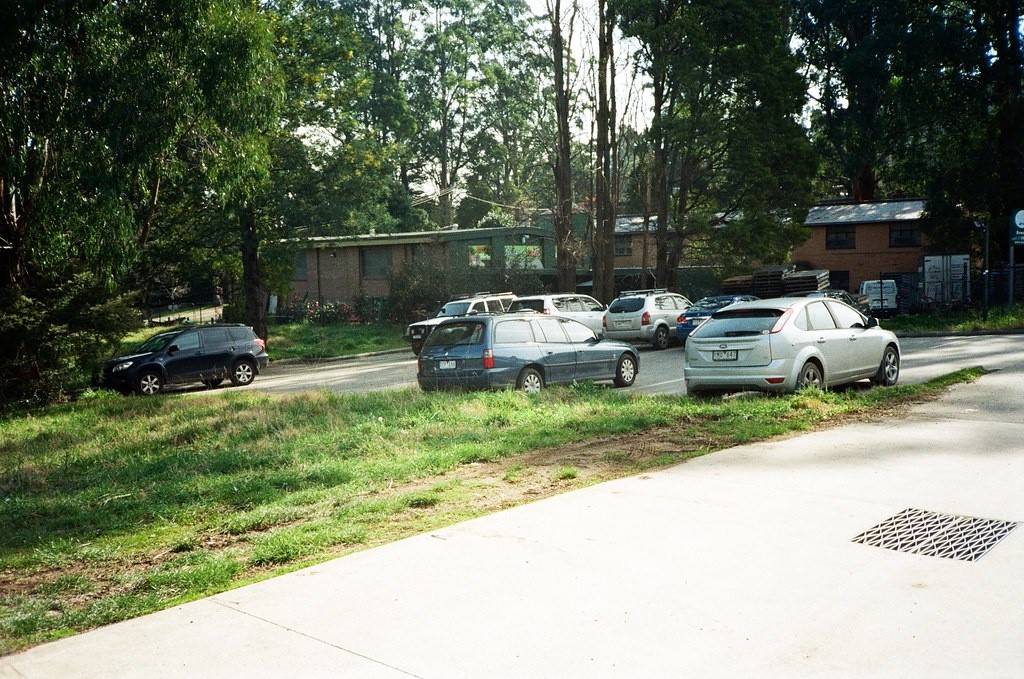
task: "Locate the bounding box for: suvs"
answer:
[416,309,641,394]
[403,291,523,358]
[102,322,268,397]
[602,288,693,349]
[504,290,608,339]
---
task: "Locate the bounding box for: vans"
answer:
[860,280,900,318]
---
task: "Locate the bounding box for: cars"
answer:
[683,297,901,401]
[783,290,873,319]
[676,294,762,347]
